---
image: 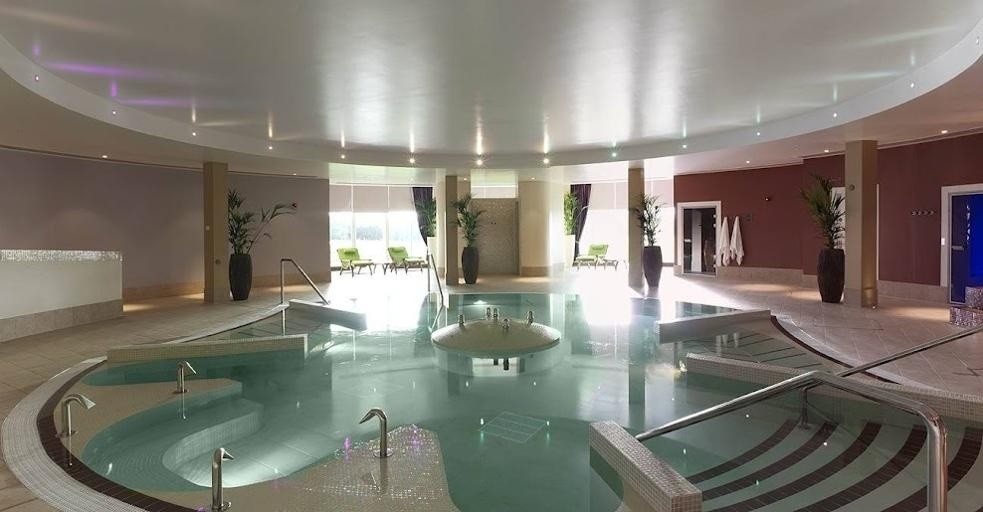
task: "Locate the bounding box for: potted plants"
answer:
[447,190,489,284]
[792,164,846,304]
[560,190,587,269]
[630,191,667,288]
[227,192,297,302]
[415,195,436,269]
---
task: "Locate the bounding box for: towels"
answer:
[715,216,730,267]
[728,215,744,266]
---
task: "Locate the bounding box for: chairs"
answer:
[386,246,425,273]
[576,240,611,269]
[337,248,377,276]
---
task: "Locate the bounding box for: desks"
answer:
[603,256,628,271]
[382,261,398,276]
